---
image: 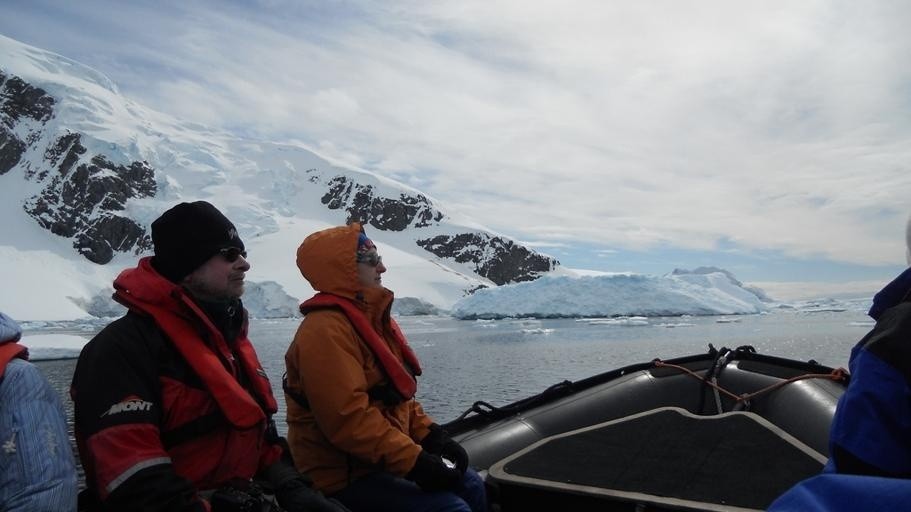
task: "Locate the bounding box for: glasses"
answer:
[220,247,247,263]
[358,256,383,266]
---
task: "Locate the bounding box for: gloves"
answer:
[405,428,468,497]
[287,486,349,511]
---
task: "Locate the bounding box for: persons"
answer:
[281,221,486,511]
[71,200,345,512]
[768,214,911,512]
[0,311,79,512]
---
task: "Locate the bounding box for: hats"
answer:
[358,233,376,260]
[151,201,245,278]
[0,311,21,342]
